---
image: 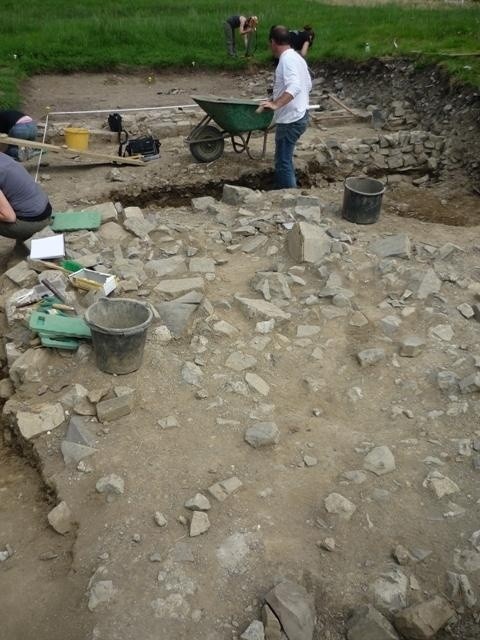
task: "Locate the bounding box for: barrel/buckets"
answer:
[85,296,152,375]
[343,163,389,225]
[63,127,89,151]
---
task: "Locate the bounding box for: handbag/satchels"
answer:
[107,113,122,132]
[126,138,161,157]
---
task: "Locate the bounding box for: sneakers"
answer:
[18,145,34,161]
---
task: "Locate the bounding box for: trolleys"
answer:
[182,93,322,163]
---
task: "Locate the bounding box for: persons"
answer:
[0,108,39,162]
[0,151,54,242]
[257,23,314,192]
[223,15,258,58]
[271,24,316,82]
